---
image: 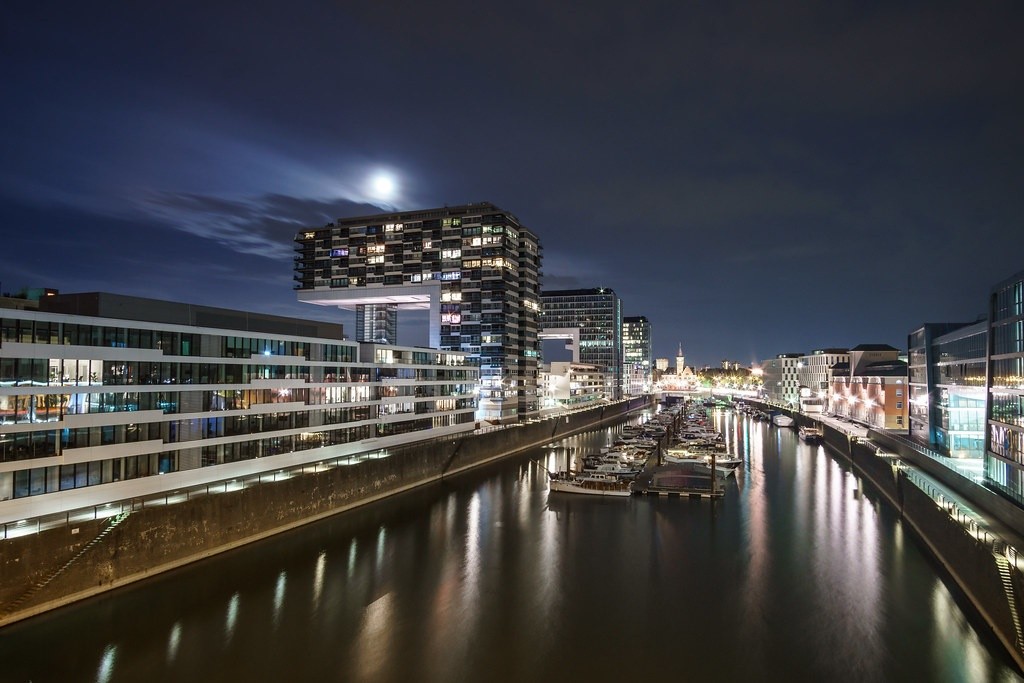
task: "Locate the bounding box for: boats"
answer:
[798,421,824,442]
[546,396,769,498]
